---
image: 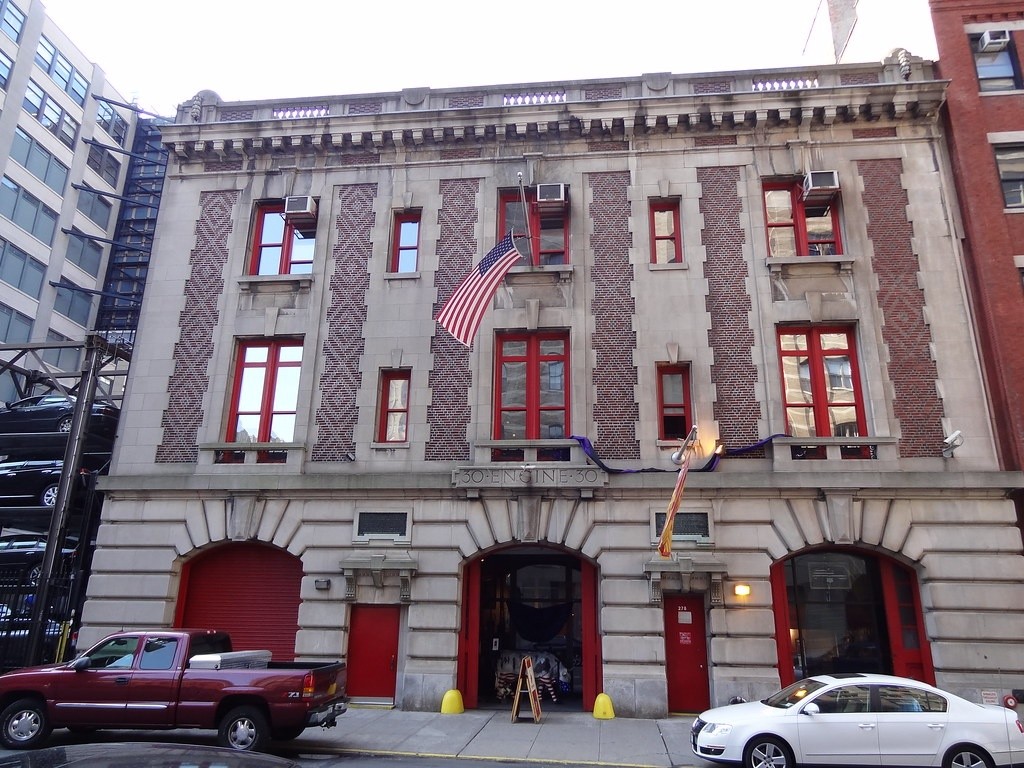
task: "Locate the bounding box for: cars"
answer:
[689,671,1024,768]
[805,640,893,675]
[0,393,121,676]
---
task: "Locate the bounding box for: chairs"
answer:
[837,694,923,712]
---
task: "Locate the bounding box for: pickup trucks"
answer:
[0,627,348,750]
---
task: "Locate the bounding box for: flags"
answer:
[657,450,690,558]
[435,227,524,348]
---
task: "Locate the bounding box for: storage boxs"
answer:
[189,650,272,669]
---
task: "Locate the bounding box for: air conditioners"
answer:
[537,183,565,211]
[802,170,839,201]
[285,196,317,217]
[977,28,1010,52]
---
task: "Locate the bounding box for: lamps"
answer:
[315,579,331,589]
